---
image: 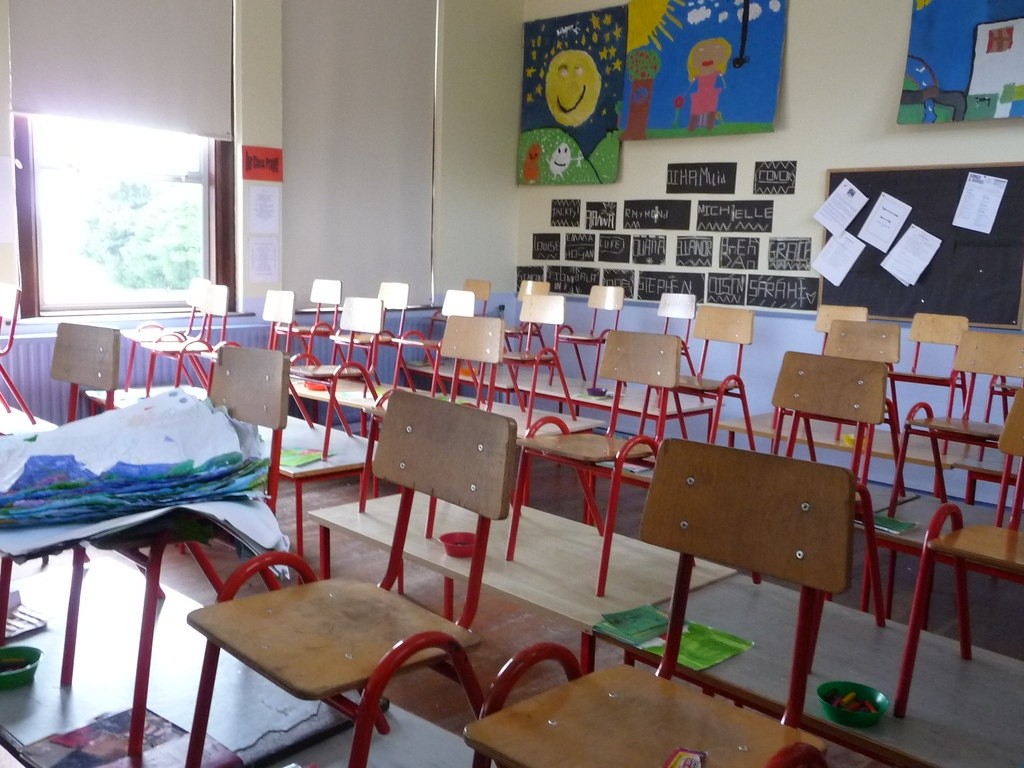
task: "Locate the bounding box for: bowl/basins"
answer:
[816,680,889,728]
[0,646,45,689]
[306,381,327,391]
[588,388,607,396]
[438,532,475,557]
[459,368,478,376]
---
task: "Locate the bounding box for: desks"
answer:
[589,412,1022,613]
[92,315,968,589]
[593,571,1024,768]
[308,490,737,680]
[1,541,500,768]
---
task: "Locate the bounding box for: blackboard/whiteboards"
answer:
[816,161,1024,331]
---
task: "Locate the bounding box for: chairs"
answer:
[0,321,122,688]
[60,345,289,758]
[184,389,519,768]
[0,288,36,426]
[119,276,214,398]
[462,437,854,768]
[139,282,240,400]
[197,272,1024,723]
